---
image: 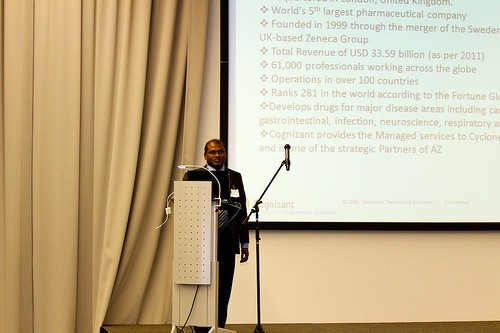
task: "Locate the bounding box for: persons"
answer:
[182,139,249,333]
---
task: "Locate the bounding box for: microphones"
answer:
[284,144,290,171]
[177,165,221,197]
